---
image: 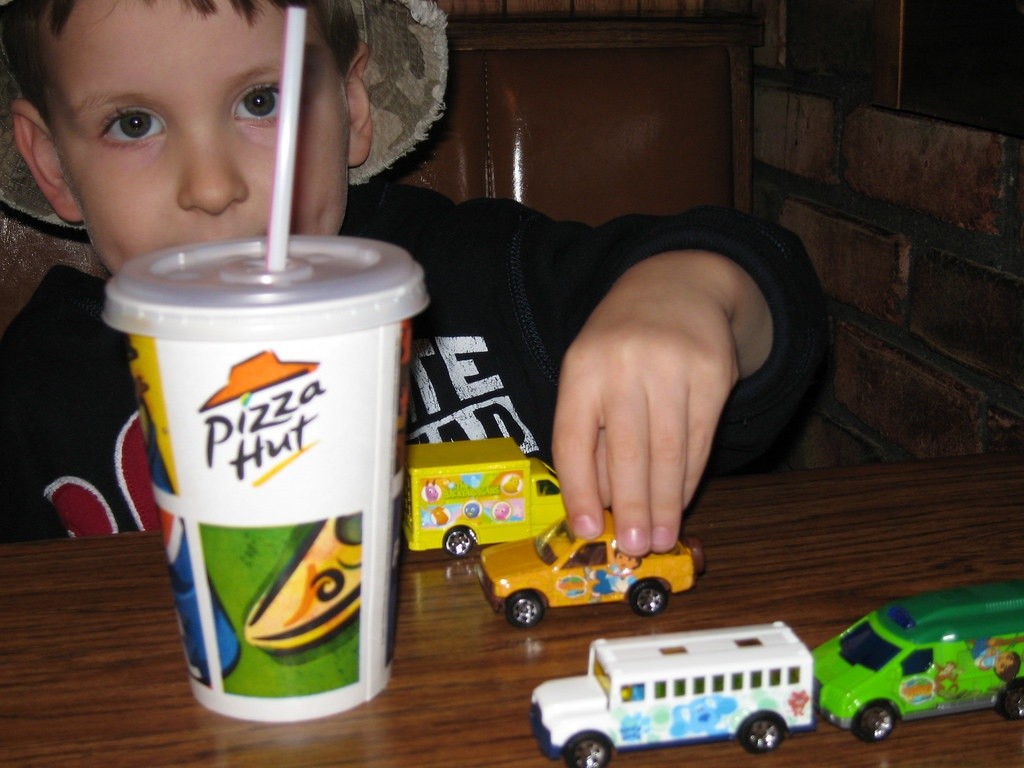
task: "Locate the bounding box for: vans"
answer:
[809,579,1024,743]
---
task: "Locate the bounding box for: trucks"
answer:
[403,436,567,557]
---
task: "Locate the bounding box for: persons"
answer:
[0,0,830,557]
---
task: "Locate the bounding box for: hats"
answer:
[0,0,449,228]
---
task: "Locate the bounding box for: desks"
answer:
[0,449,1024,768]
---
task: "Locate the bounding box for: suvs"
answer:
[478,509,705,628]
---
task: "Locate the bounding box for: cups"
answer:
[101,233,431,724]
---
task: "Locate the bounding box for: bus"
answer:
[528,622,816,768]
